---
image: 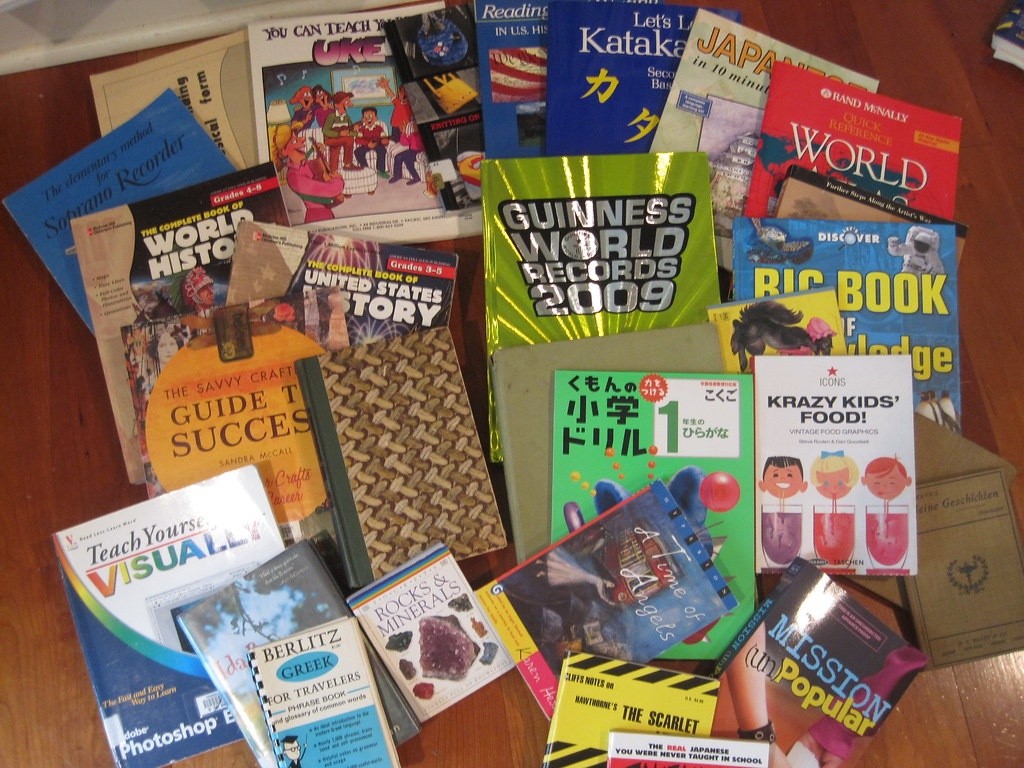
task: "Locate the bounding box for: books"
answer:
[1,4,971,768]
[990,0,1024,71]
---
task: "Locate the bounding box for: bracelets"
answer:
[738,722,776,745]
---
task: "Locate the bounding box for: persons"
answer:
[787,647,926,768]
[725,620,789,767]
